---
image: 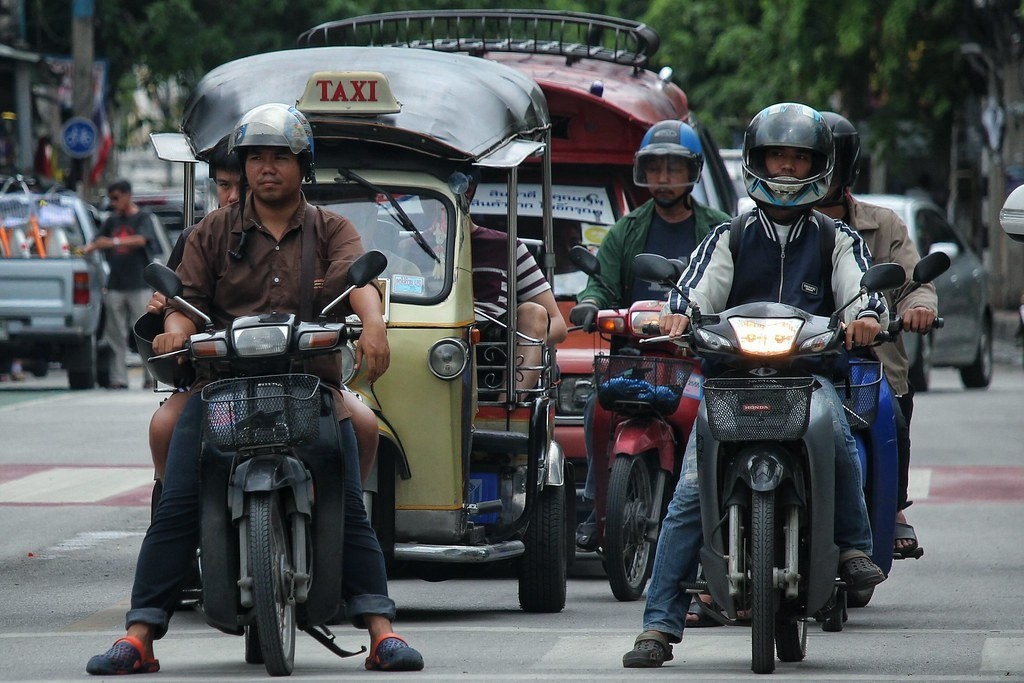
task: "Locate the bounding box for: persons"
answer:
[74,179,163,390]
[318,166,568,404]
[85,102,425,676]
[575,102,938,666]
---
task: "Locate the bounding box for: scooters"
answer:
[141,250,387,676]
[564,243,950,674]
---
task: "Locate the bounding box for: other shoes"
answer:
[575,521,600,551]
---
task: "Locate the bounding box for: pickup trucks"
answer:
[1,193,116,391]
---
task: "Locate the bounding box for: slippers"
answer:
[685,602,724,628]
[86,636,161,674]
[365,631,425,671]
[893,522,918,553]
[623,632,673,667]
[841,549,885,591]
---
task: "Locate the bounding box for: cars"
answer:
[297,7,740,525]
[853,193,997,394]
[95,183,216,254]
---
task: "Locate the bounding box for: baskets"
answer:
[838,361,883,428]
[703,377,815,440]
[593,353,694,417]
[200,373,321,449]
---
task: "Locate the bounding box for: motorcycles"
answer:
[148,51,579,617]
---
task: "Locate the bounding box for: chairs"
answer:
[470,231,553,409]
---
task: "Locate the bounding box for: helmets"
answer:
[633,120,704,187]
[820,111,860,187]
[741,102,835,207]
[133,311,192,388]
[227,103,314,185]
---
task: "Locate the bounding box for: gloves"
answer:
[569,302,599,334]
